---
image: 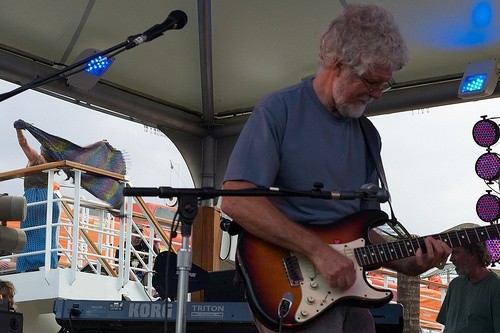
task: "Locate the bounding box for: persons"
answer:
[221,3,452,333]
[114,225,159,271]
[15,119,60,274]
[0,280,20,313]
[437,223,500,333]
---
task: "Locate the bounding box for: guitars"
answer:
[234,209,500,333]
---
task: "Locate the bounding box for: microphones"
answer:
[362,182,389,203]
[128,9,189,50]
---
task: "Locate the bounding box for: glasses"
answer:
[357,73,392,97]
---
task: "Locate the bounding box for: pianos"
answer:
[54,296,260,333]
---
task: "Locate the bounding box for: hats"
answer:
[132,222,143,230]
